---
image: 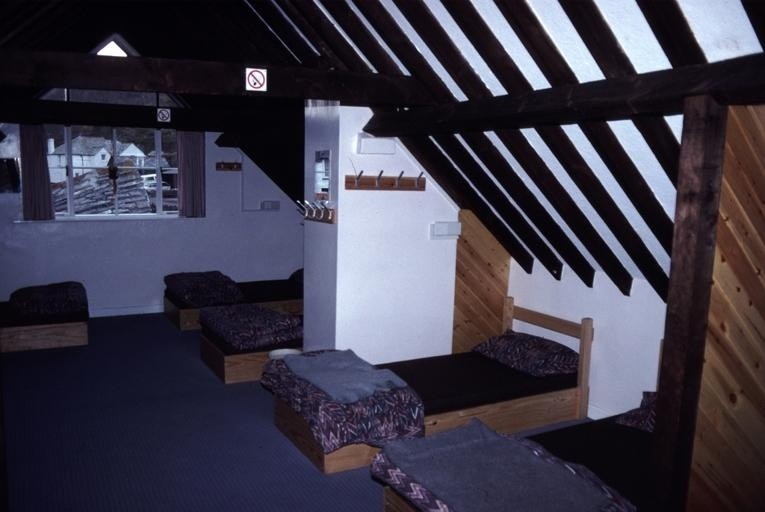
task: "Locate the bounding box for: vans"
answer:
[141,174,178,212]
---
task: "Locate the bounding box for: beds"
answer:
[197,300,304,388]
[264,297,595,480]
[162,267,304,333]
[0,280,89,354]
[373,391,691,512]
